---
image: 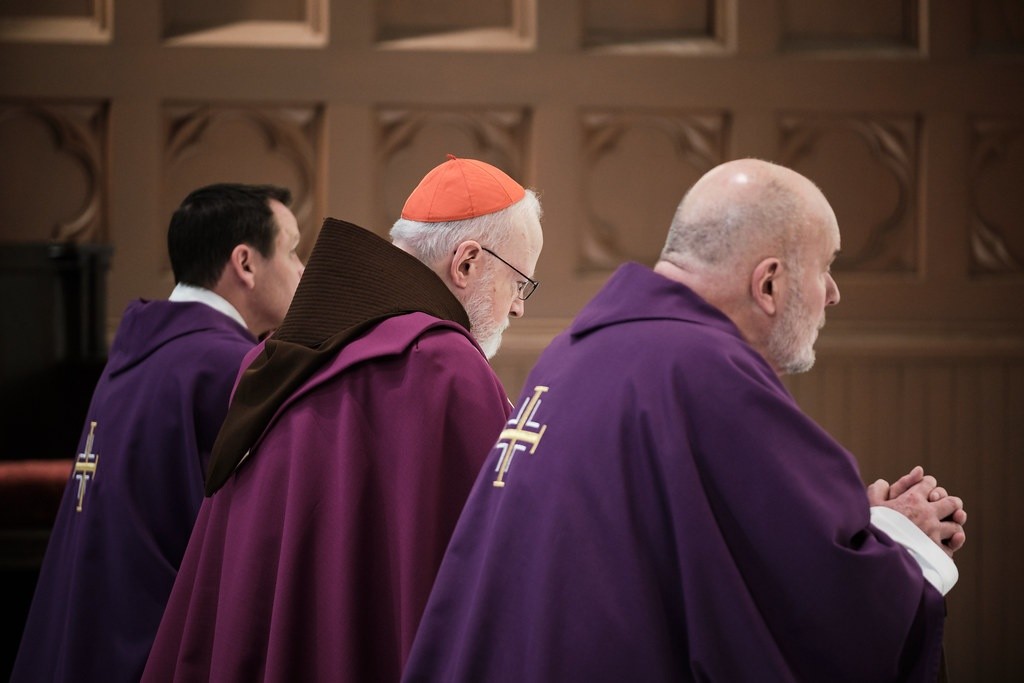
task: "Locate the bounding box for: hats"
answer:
[401,154,526,224]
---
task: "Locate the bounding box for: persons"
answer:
[401,157,966,683]
[139,153,543,683]
[8,180,308,683]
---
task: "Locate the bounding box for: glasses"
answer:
[454,247,538,301]
